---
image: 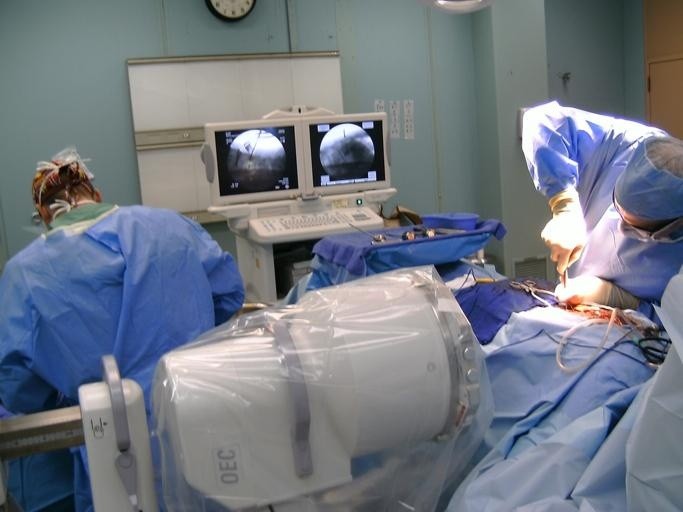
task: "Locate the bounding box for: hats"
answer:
[616,135,682,220]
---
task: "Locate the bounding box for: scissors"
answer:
[509,280,559,306]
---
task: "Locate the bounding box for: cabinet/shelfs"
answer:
[643,0,683,141]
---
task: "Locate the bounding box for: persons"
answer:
[521,99,683,307]
[0,145,244,512]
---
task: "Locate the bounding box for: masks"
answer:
[612,187,683,244]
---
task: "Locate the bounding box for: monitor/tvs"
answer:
[204,119,306,207]
[305,111,391,200]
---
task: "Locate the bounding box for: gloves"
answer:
[556,274,639,310]
[541,188,586,272]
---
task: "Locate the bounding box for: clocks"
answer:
[205,0,257,23]
